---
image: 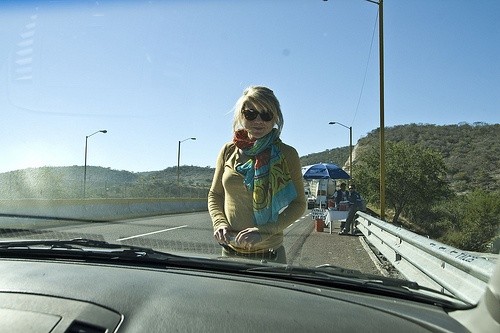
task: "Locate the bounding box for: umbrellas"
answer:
[301,162,352,208]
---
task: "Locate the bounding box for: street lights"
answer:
[176,137,196,201]
[83,130,107,199]
[328,121,352,196]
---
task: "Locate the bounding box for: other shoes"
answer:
[338,227,350,234]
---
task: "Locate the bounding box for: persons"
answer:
[335,183,350,211]
[338,182,363,234]
[207,86,307,263]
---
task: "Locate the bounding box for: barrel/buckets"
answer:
[328,199,335,210]
[314,218,324,233]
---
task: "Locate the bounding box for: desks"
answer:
[325,209,354,234]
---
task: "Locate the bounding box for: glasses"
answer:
[241,109,274,122]
